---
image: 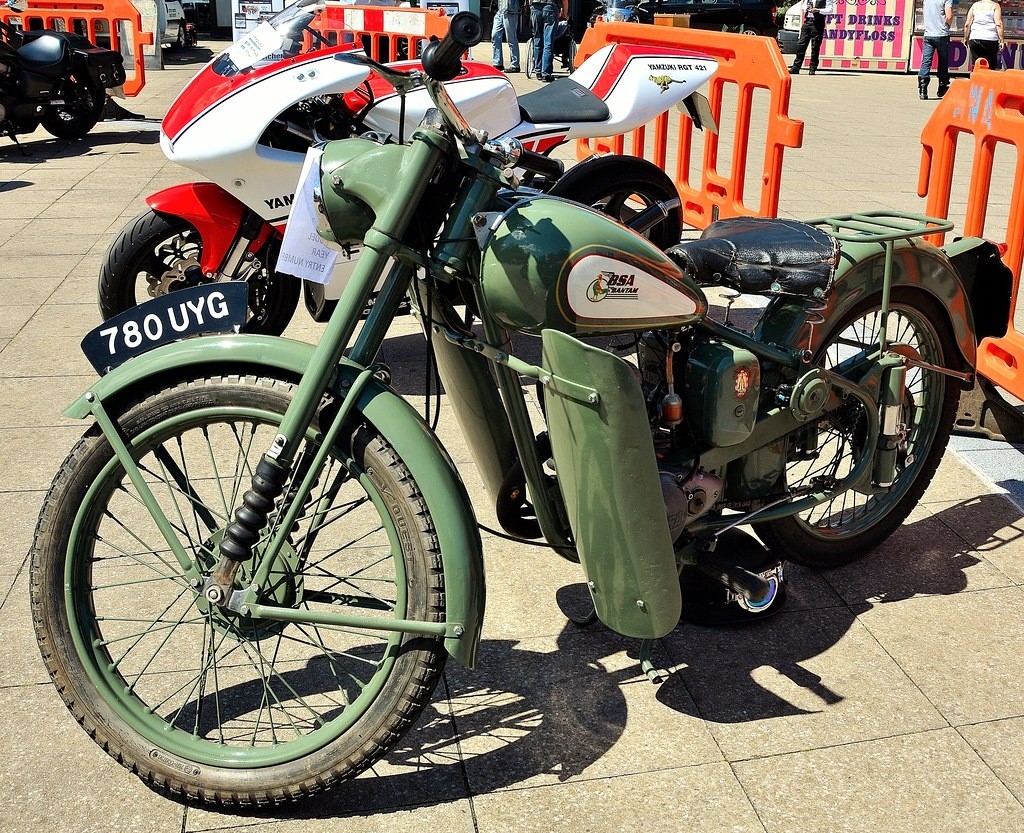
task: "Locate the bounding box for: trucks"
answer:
[774,0,1024,55]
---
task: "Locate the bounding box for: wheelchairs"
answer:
[525,28,577,81]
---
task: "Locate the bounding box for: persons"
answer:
[918,0,953,100]
[963,1,1005,72]
[788,0,833,75]
[490,0,563,83]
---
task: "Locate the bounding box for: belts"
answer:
[807,17,814,21]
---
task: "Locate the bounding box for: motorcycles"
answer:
[22,9,1018,814]
[0,20,126,156]
[587,0,651,26]
[99,0,720,365]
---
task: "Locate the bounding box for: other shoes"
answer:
[493,65,504,71]
[809,69,815,75]
[504,67,520,73]
[536,73,542,79]
[542,76,554,82]
[788,66,799,74]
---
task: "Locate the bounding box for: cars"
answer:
[589,0,779,48]
[161,1,188,50]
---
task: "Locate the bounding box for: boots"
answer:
[937,82,949,96]
[918,80,929,99]
